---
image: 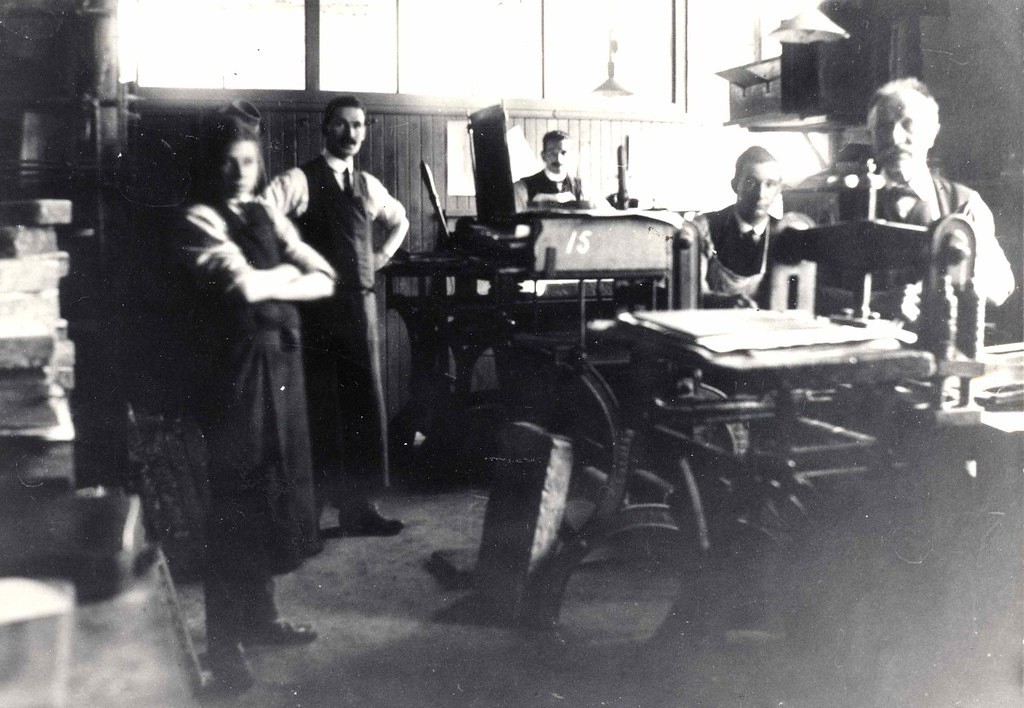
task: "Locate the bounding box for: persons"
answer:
[840,78,1016,333]
[691,144,818,312]
[179,96,413,688]
[513,130,598,213]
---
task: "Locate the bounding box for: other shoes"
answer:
[241,617,316,646]
[210,639,254,694]
[339,503,405,536]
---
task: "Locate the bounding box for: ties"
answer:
[343,167,351,196]
[742,229,756,264]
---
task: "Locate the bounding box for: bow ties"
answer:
[890,187,917,201]
